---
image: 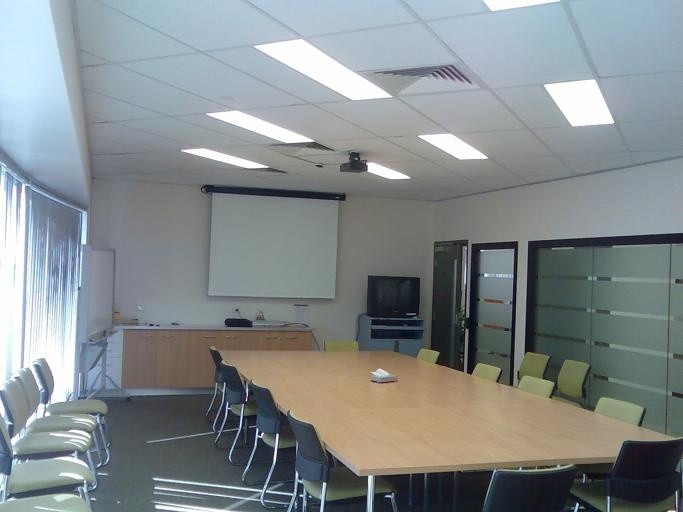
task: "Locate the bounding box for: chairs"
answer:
[323,339,360,352]
[205,344,397,512]
[0,357,111,512]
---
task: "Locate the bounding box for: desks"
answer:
[217,348,683,512]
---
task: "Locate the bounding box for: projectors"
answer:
[340,163,367,173]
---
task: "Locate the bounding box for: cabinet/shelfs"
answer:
[120,327,312,397]
[79,326,124,398]
[357,312,424,358]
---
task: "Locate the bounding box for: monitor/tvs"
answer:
[367,276,420,318]
